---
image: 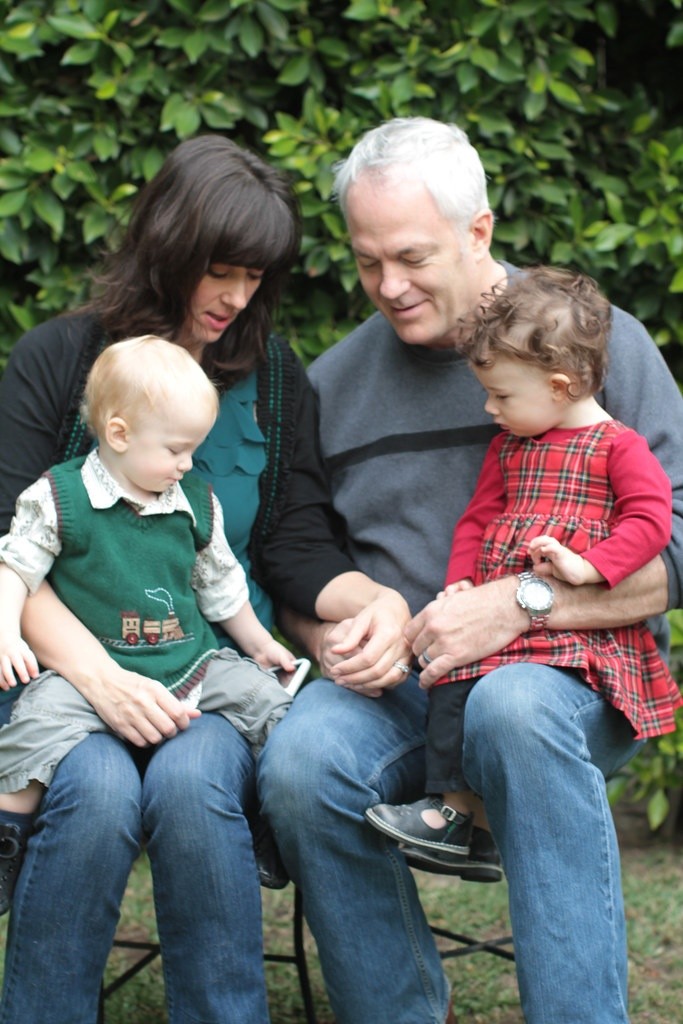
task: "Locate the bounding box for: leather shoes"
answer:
[398,825,503,882]
[364,796,475,855]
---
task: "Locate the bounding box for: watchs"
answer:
[514,570,553,632]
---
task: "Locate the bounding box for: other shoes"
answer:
[255,817,290,889]
[0,824,31,915]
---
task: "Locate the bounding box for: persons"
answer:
[257,115,683,1023]
[364,268,672,883]
[0,135,411,1024]
[0,334,291,916]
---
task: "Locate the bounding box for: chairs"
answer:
[99,886,516,1024]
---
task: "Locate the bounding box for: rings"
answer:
[393,662,410,673]
[422,650,431,664]
